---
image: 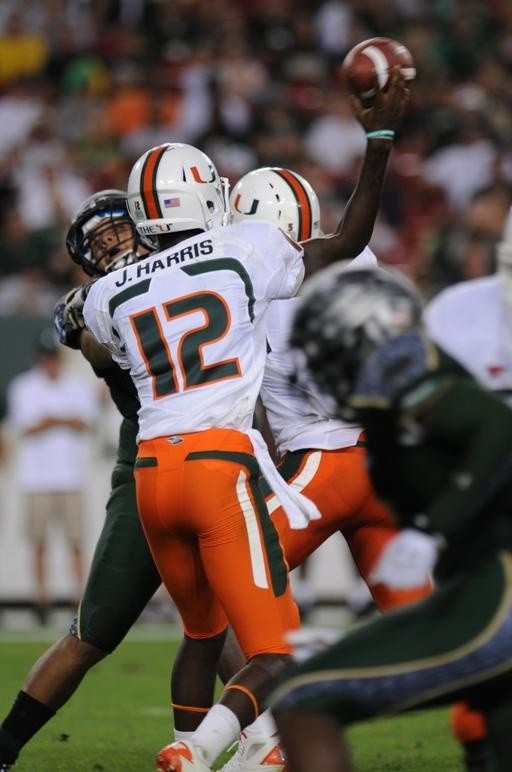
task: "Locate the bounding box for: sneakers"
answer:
[155,739,209,772]
[214,729,289,772]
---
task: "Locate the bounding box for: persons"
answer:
[1,1,512,410]
[266,256,509,770]
[214,161,494,771]
[0,187,250,771]
[1,322,107,625]
[84,58,416,772]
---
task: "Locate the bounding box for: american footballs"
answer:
[342,38,416,98]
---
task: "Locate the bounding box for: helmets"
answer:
[126,142,229,248]
[498,206,512,308]
[230,167,321,242]
[288,268,444,421]
[66,189,150,271]
